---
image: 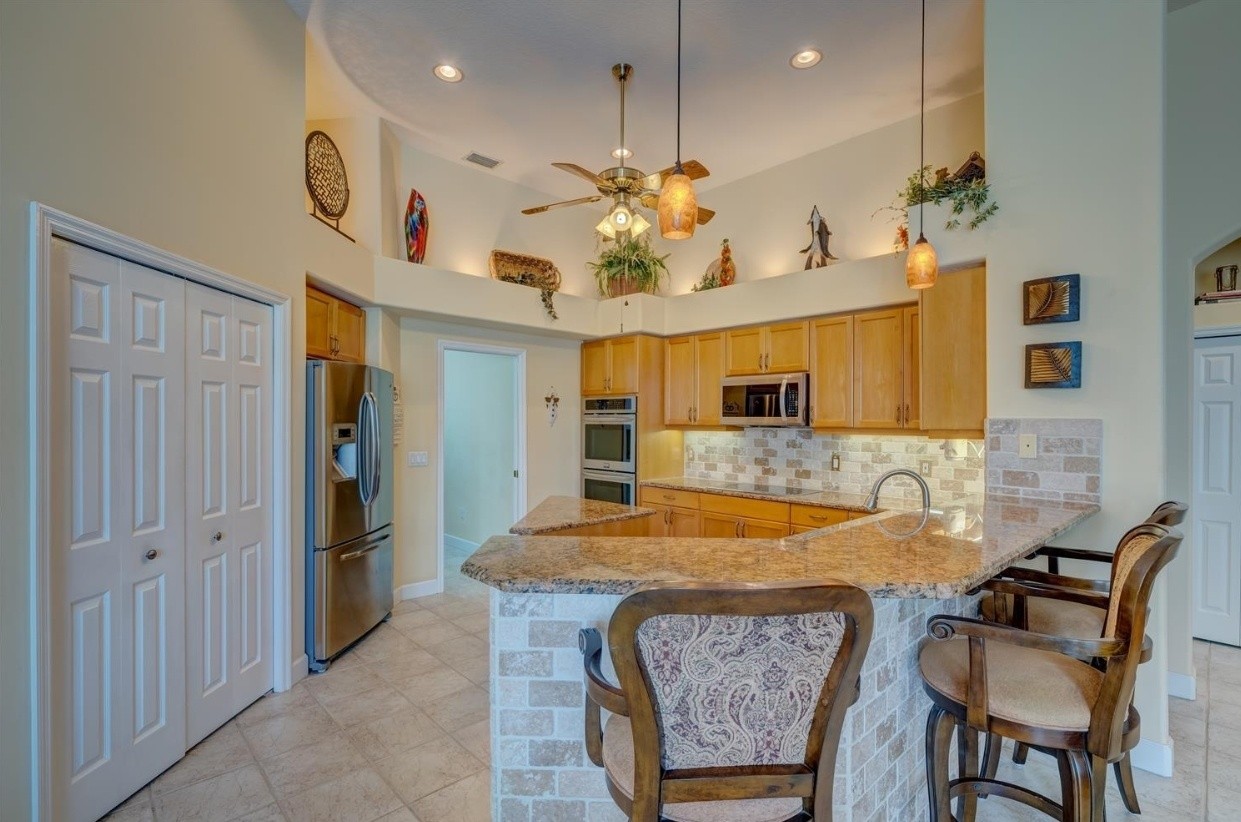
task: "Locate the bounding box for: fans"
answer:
[521,64,715,225]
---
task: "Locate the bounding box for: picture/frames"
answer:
[1023,274,1080,324]
[1025,341,1081,389]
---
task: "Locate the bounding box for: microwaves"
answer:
[719,372,810,428]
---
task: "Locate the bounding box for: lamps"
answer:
[597,203,652,239]
[905,0,938,288]
[657,0,698,240]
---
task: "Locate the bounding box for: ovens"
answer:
[580,396,637,505]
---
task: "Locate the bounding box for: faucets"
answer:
[872,511,929,541]
[860,468,931,510]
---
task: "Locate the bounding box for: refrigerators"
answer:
[305,357,396,675]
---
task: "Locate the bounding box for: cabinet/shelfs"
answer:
[725,320,809,377]
[640,485,699,538]
[809,317,852,426]
[305,286,366,366]
[790,504,871,536]
[700,493,790,539]
[664,332,724,427]
[579,335,641,395]
[920,263,986,438]
[854,309,920,431]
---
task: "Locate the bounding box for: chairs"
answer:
[917,527,1182,822]
[978,499,1188,815]
[579,577,874,822]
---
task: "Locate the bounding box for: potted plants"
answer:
[584,238,670,298]
